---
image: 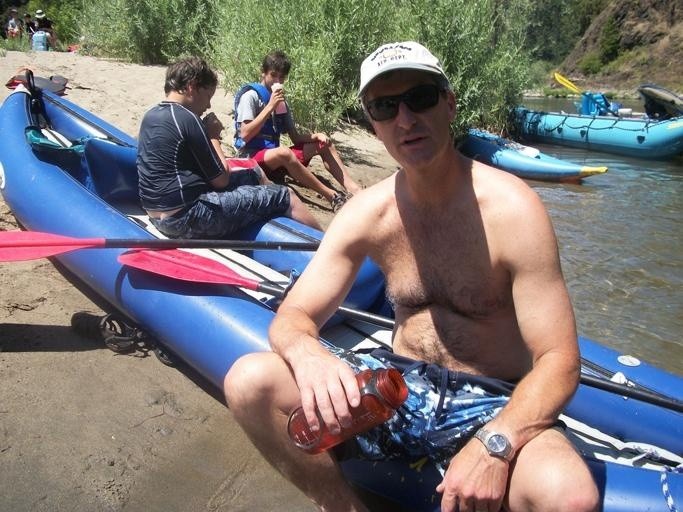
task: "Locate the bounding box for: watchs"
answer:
[475,428,516,462]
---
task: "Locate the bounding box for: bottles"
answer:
[270,83,288,115]
[285,366,409,455]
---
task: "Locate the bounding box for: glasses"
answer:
[362,81,440,121]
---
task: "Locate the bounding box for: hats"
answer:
[356,41,448,99]
[35,9,45,18]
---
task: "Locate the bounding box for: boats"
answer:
[459,128,607,183]
[511,90,683,159]
[0,74,683,512]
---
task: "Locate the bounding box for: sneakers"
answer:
[330,190,353,214]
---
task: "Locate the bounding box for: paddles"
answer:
[115,247,682,413]
[553,71,621,118]
[0,229,322,263]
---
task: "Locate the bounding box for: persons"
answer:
[232,51,362,214]
[135,56,326,240]
[224,41,602,512]
[5,9,57,49]
